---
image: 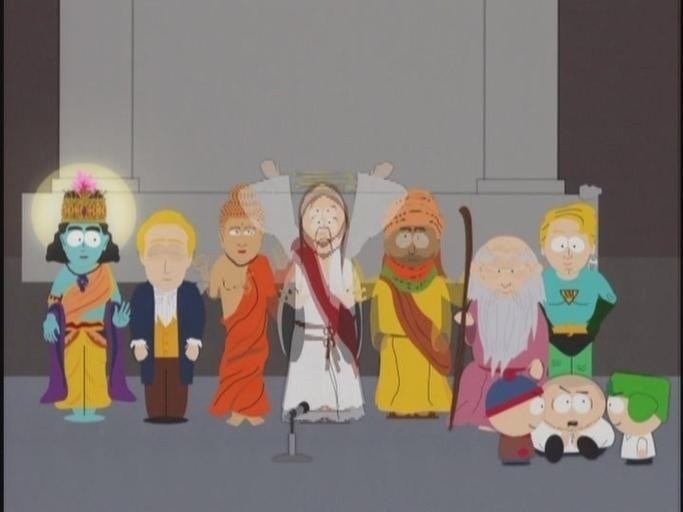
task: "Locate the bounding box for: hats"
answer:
[484,375,545,417]
[383,189,443,244]
[60,185,108,222]
[605,371,670,426]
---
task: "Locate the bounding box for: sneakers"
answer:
[545,434,601,463]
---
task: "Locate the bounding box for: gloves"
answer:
[586,296,614,336]
